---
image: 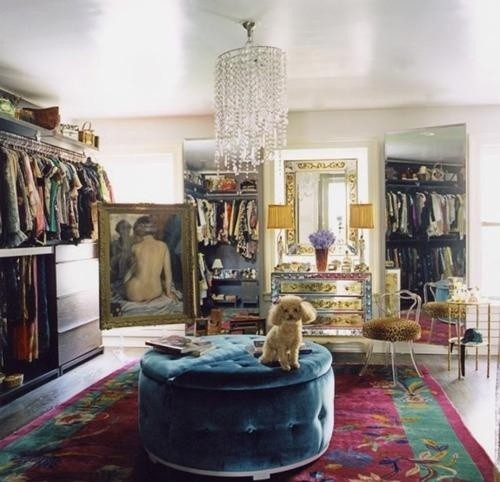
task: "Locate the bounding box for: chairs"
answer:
[358,290,423,385]
[423,282,466,344]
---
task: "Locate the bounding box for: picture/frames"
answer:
[97,203,197,329]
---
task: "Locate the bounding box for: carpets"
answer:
[0,358,500,482]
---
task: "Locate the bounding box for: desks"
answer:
[448,299,493,380]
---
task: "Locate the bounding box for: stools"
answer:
[137,334,335,476]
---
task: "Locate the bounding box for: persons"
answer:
[110,216,176,302]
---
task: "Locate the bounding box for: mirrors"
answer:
[283,157,358,255]
[383,123,470,345]
[181,135,265,333]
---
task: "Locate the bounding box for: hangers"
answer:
[0,130,83,164]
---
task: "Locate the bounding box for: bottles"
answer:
[447,275,480,303]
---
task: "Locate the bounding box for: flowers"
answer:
[309,230,335,249]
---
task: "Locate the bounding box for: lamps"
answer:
[267,204,293,254]
[215,21,288,177]
[349,203,374,258]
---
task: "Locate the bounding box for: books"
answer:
[144,334,216,357]
[250,339,312,357]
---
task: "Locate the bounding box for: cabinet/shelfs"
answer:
[0,241,104,402]
[271,271,372,337]
[184,169,260,324]
[386,157,466,309]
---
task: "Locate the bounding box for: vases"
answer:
[315,249,329,272]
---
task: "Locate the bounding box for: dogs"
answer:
[259,295,316,371]
